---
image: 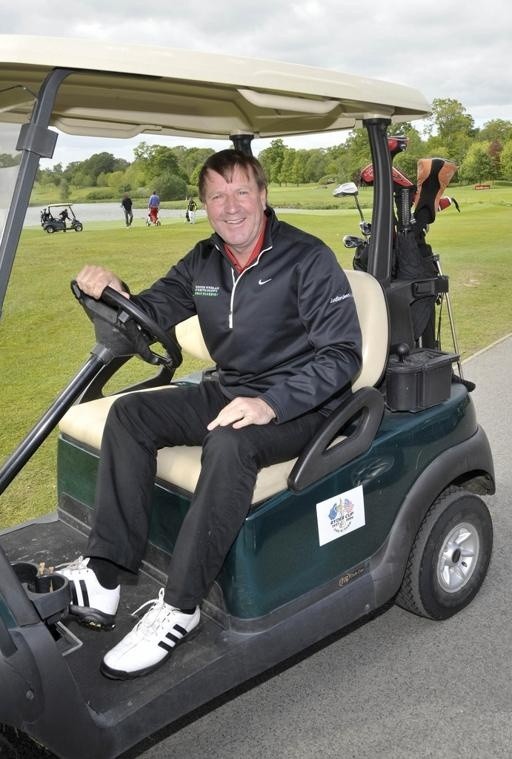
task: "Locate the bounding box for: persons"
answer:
[40,209,72,223]
[187,197,197,225]
[39,150,364,680]
[148,190,160,224]
[120,192,134,227]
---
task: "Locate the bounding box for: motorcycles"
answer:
[42,202,81,235]
[0,61,495,757]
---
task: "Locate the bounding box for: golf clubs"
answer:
[332,133,456,250]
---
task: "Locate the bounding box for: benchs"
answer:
[50,265,388,523]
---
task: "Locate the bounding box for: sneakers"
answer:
[53,557,120,632]
[101,596,203,682]
